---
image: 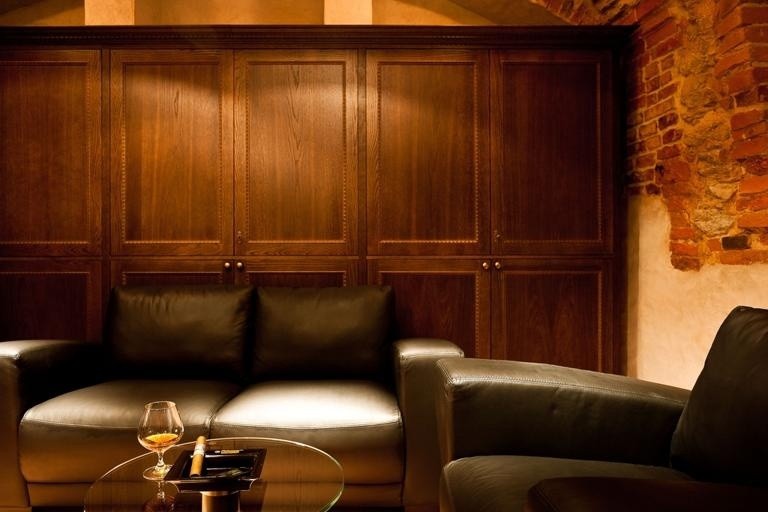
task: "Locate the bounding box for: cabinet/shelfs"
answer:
[362,25,625,255]
[366,258,629,375]
[0,25,107,258]
[106,25,362,256]
[107,255,365,289]
[0,258,110,343]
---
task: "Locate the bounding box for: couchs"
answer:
[1,288,465,512]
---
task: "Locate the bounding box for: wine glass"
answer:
[136,400,185,480]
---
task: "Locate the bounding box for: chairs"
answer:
[430,305,768,512]
